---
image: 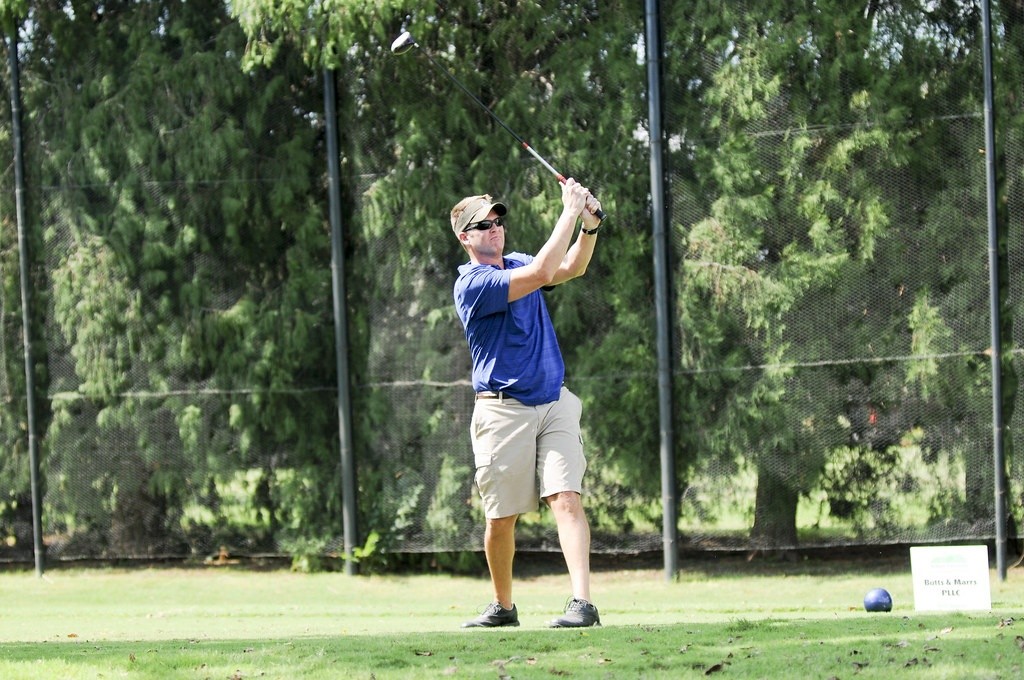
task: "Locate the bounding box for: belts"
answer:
[477,390,515,400]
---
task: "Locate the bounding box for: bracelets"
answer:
[582,226,598,235]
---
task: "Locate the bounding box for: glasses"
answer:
[463,217,504,232]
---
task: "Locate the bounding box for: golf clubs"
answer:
[391,31,608,223]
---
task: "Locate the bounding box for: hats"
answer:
[455,193,508,238]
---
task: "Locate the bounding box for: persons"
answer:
[451,177,602,628]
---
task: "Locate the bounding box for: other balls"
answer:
[864,587,892,612]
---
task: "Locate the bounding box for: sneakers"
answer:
[548,595,599,628]
[461,602,520,627]
